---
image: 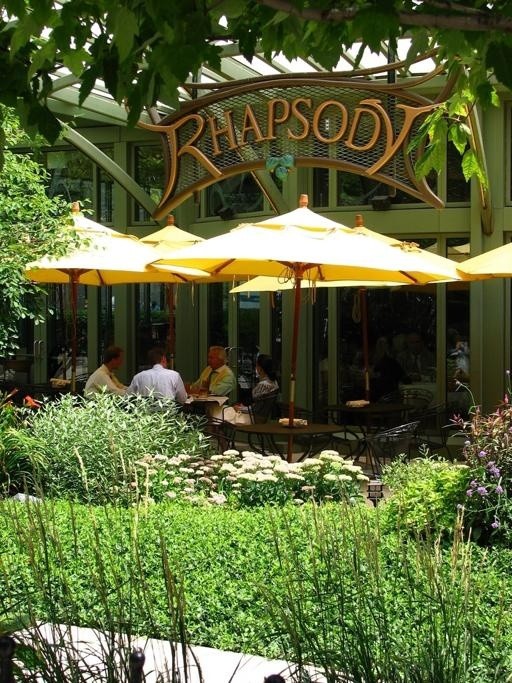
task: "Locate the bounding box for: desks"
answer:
[235,401,415,462]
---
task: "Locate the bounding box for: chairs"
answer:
[129,389,282,454]
[356,387,453,477]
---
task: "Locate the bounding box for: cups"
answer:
[190,376,209,400]
